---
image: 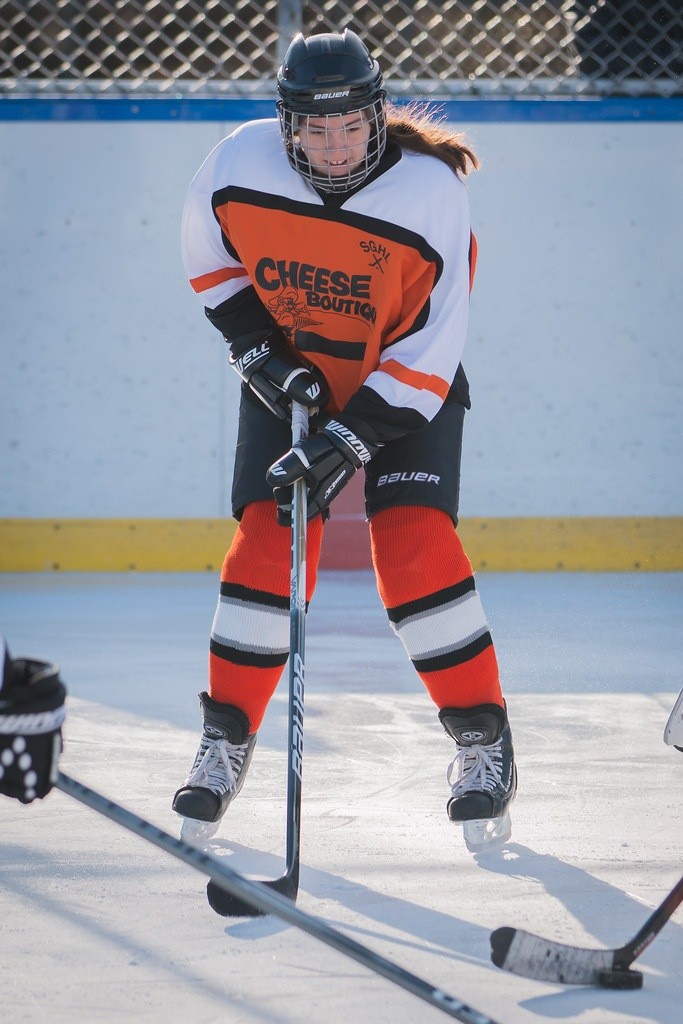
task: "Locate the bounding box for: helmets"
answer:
[275,27,389,197]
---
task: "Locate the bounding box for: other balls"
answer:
[600,970,644,990]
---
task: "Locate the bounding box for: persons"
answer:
[173,29,518,851]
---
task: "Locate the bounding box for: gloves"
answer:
[266,391,405,529]
[2,653,68,804]
[202,284,330,425]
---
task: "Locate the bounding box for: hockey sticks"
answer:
[205,396,303,917]
[55,771,497,1024]
[490,875,683,986]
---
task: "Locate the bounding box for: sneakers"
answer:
[170,692,257,841]
[440,706,520,854]
